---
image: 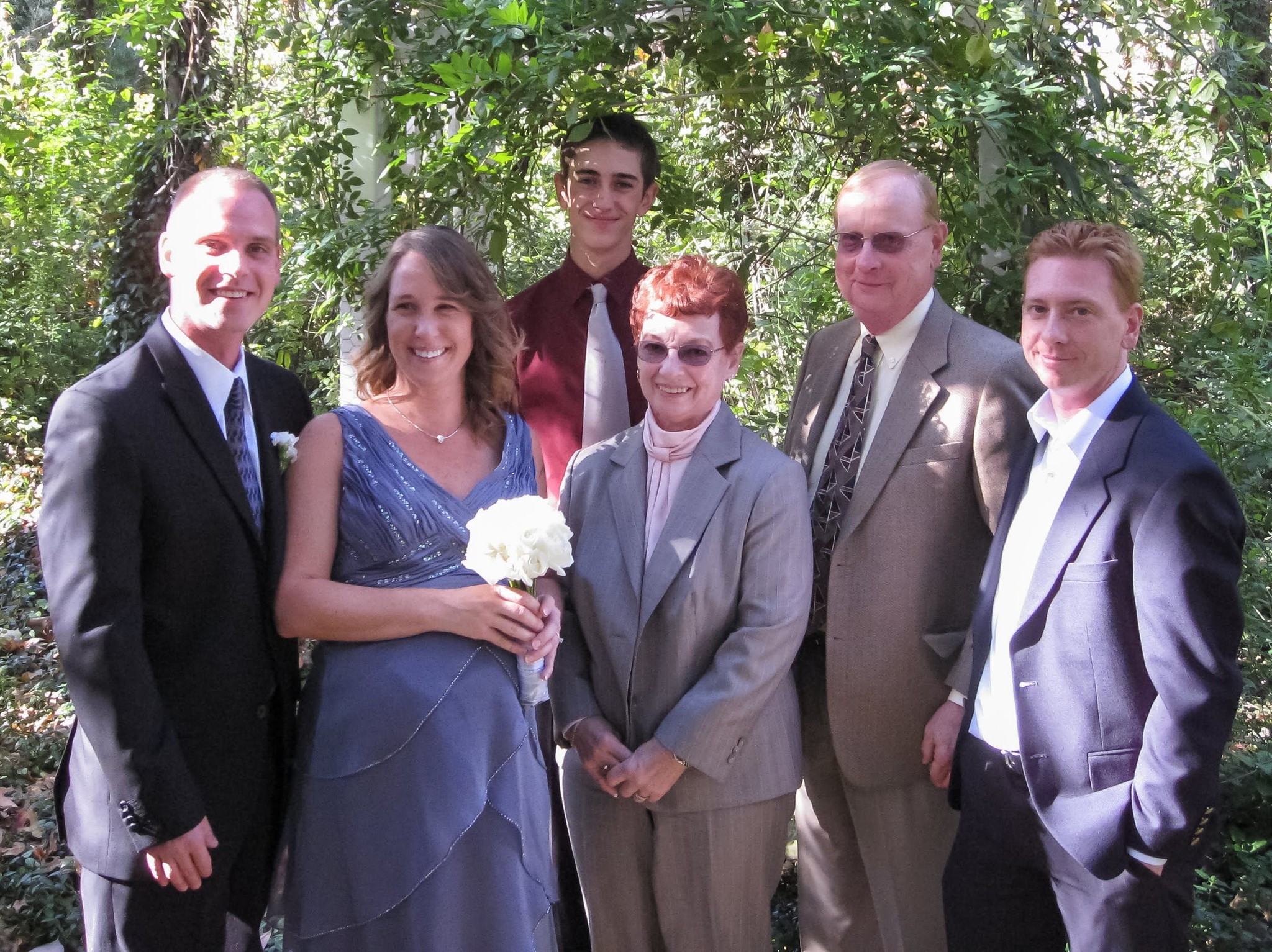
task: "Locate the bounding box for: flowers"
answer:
[461,495,574,702]
[269,430,300,475]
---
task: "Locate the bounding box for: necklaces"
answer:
[387,388,470,443]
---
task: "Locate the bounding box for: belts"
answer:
[967,736,1027,777]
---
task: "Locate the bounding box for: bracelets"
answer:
[674,754,690,767]
[568,717,588,746]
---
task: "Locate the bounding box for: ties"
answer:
[223,377,266,540]
[800,335,879,654]
[581,282,631,449]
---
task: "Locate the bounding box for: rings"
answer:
[556,633,560,639]
[635,793,648,803]
[603,765,608,770]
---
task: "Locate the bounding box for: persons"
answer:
[939,219,1247,952]
[275,224,564,952]
[782,160,1049,952]
[547,253,813,952]
[37,168,315,952]
[504,113,662,952]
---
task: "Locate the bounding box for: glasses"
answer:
[828,225,932,254]
[633,339,728,367]
